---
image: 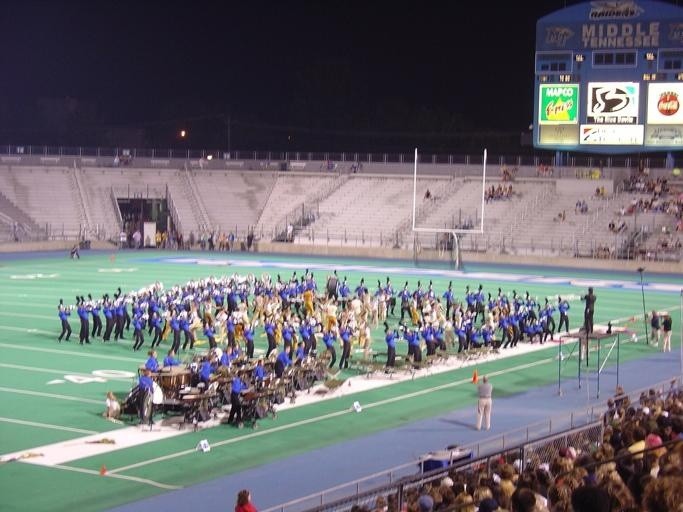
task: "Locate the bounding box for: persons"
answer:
[56,264,599,430]
[233,490,258,512]
[343,378,683,511]
[647,310,660,348]
[117,227,255,251]
[438,156,682,265]
[69,240,81,258]
[661,312,673,355]
[287,223,296,242]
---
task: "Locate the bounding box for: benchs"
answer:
[0,163,682,262]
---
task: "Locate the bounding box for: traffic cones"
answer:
[471,369,479,385]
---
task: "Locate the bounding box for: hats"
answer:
[441,477,453,487]
[418,495,434,511]
[480,498,498,512]
